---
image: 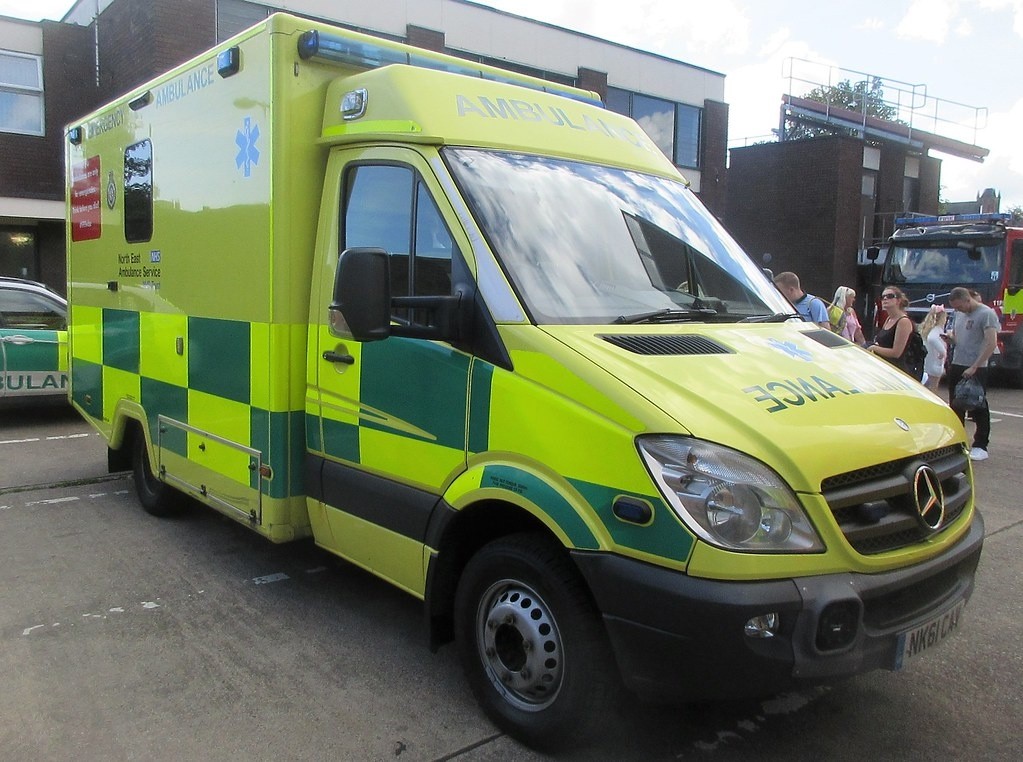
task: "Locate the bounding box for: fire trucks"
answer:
[872,212,1023,386]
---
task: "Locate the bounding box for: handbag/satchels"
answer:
[951,378,988,410]
[894,316,928,383]
[808,297,847,335]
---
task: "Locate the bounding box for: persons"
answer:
[866,285,925,384]
[939,287,1001,461]
[773,271,831,333]
[827,285,865,345]
[918,304,947,393]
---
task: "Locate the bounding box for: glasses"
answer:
[881,293,901,299]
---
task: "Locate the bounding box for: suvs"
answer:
[0,277,80,418]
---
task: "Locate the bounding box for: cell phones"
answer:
[940,334,951,339]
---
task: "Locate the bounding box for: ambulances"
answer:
[66,12,985,749]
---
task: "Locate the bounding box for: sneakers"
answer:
[970,447,988,460]
